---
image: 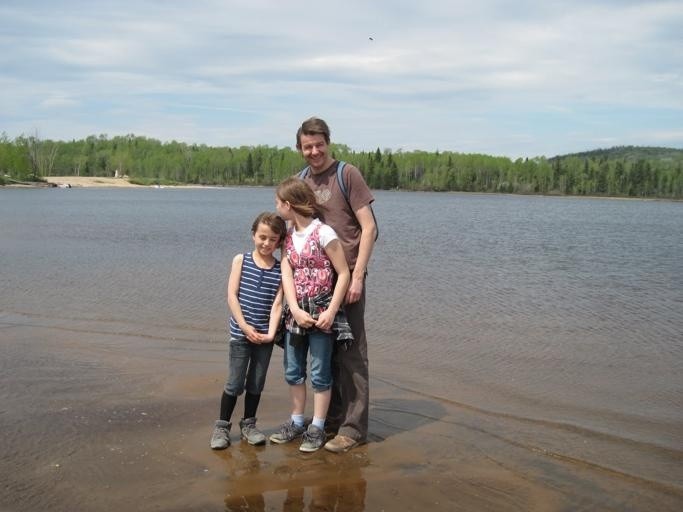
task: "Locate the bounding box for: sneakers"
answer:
[269,419,306,444]
[299,424,367,452]
[211,420,232,449]
[239,417,266,445]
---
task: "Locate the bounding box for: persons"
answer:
[286,116,377,454]
[217,442,367,511]
[210,207,284,450]
[269,177,350,453]
